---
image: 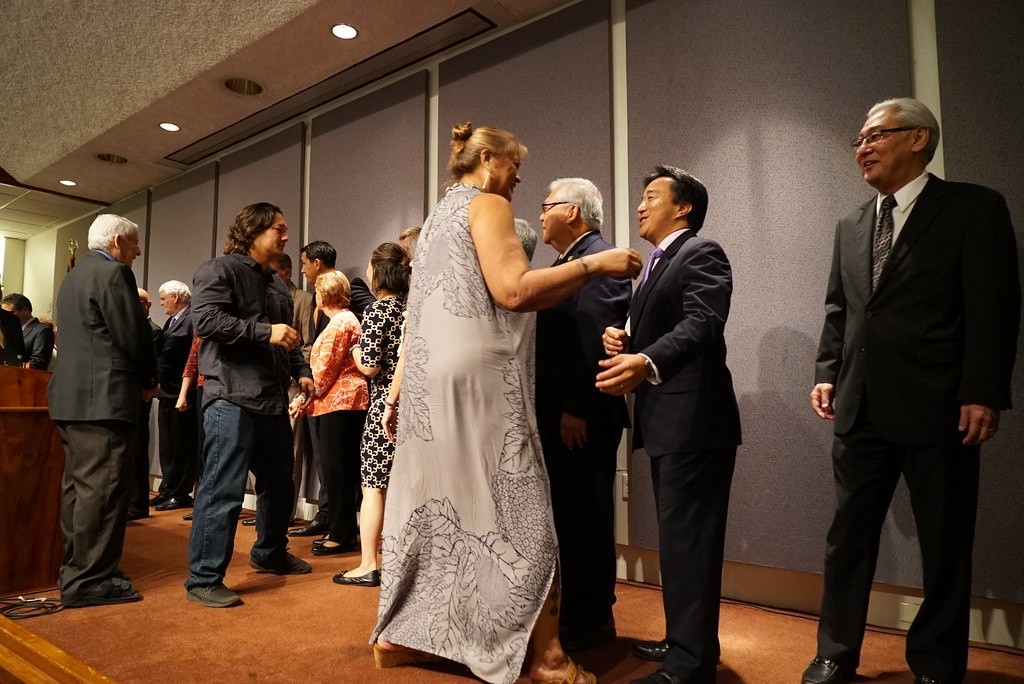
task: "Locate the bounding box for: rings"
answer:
[989,429,995,431]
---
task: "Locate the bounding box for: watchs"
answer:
[644,360,655,376]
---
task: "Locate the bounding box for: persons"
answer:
[288,241,378,536]
[332,242,411,588]
[242,253,315,526]
[0,286,26,367]
[368,121,643,684]
[127,287,164,521]
[1,293,55,370]
[148,280,205,511]
[399,226,422,259]
[514,219,537,262]
[288,270,370,556]
[596,164,742,684]
[534,176,634,648]
[801,98,1021,684]
[184,201,315,608]
[46,213,162,607]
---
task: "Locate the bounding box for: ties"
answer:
[641,248,664,293]
[313,306,319,330]
[873,196,899,292]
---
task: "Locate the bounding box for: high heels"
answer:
[374,643,442,668]
[535,655,597,684]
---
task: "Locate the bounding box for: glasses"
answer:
[851,126,920,150]
[541,199,568,213]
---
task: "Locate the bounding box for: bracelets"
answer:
[351,346,360,353]
[384,400,397,406]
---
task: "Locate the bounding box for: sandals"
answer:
[71,576,140,608]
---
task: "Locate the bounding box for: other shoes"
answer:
[333,571,379,587]
[314,536,329,544]
[312,543,351,554]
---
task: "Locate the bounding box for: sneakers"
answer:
[185,584,239,607]
[249,552,313,574]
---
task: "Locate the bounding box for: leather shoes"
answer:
[183,512,195,520]
[802,655,858,684]
[288,520,327,536]
[635,638,669,661]
[631,668,692,684]
[156,499,192,511]
[243,517,257,525]
[149,491,165,505]
